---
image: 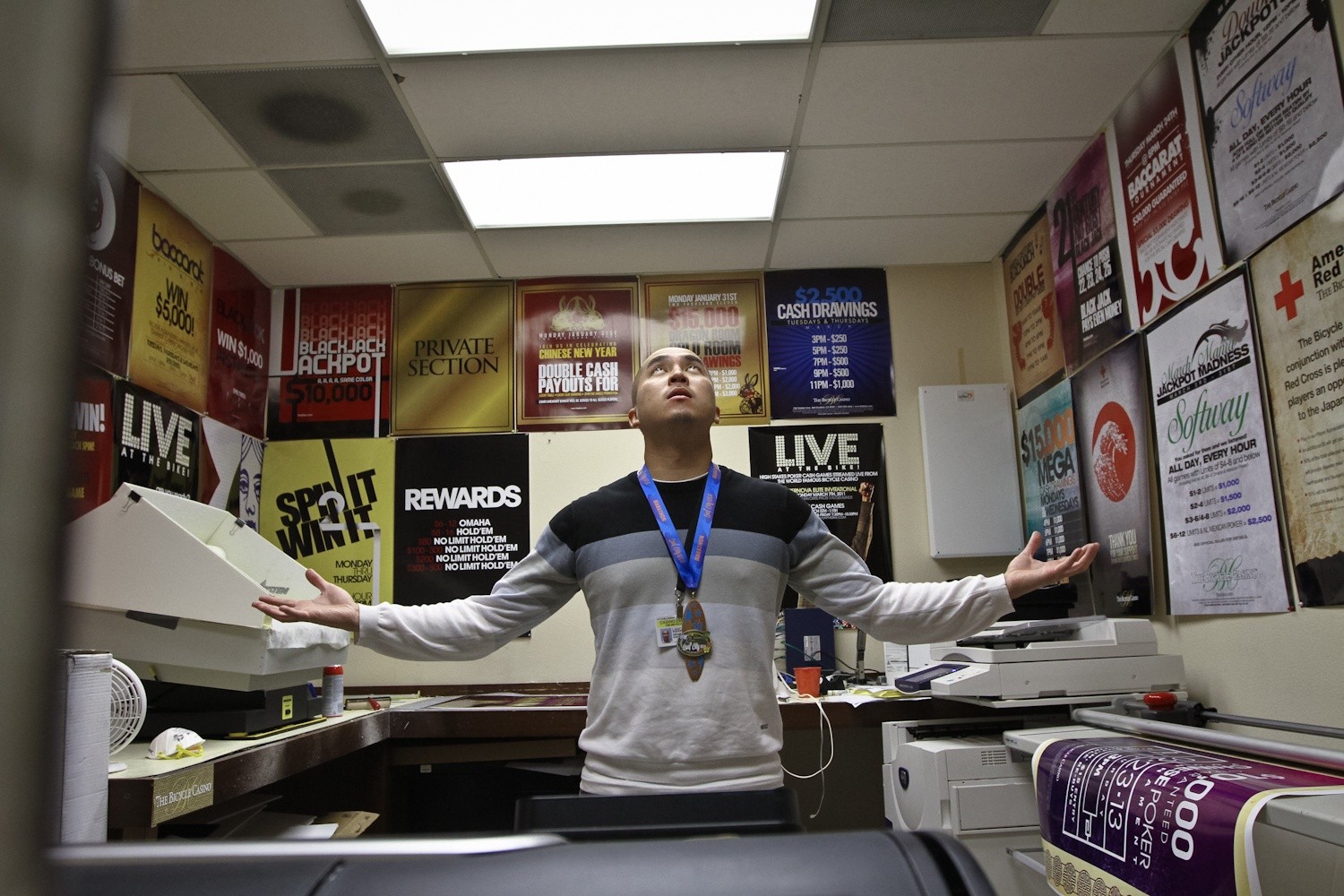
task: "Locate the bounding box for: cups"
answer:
[793,666,821,698]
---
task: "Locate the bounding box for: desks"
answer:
[114,683,957,838]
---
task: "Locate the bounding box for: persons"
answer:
[252,347,1100,792]
[662,630,671,644]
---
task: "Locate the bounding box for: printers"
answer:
[896,615,1185,702]
[881,712,1137,836]
